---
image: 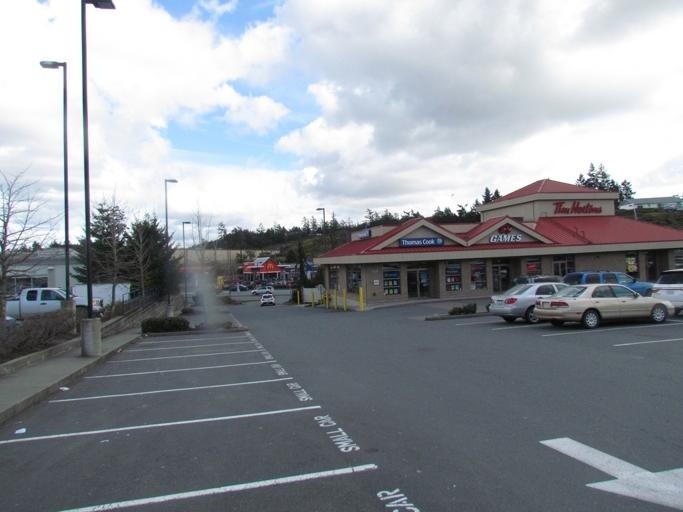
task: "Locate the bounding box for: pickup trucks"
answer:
[3,288,102,322]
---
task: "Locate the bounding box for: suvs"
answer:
[250,286,274,295]
[652,269,683,314]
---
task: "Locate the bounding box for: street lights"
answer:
[182,221,190,298]
[81,0,115,318]
[41,60,70,300]
[317,208,325,223]
[165,179,178,305]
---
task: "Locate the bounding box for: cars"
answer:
[487,283,581,324]
[230,281,255,291]
[532,283,675,329]
[563,271,653,296]
[259,294,275,306]
[513,275,562,284]
